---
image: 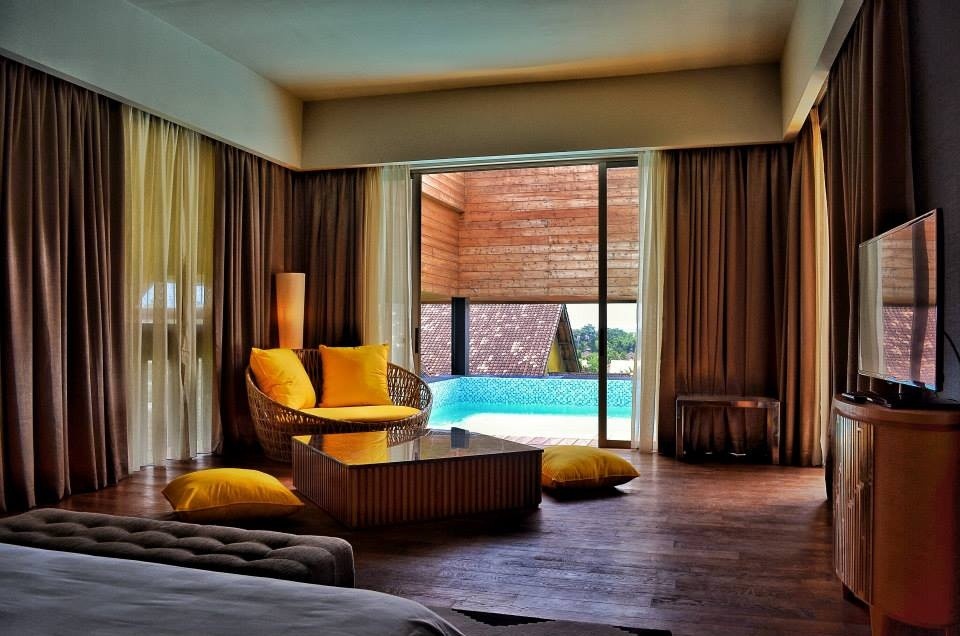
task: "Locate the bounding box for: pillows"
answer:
[318,343,394,408]
[250,347,317,411]
[541,445,641,496]
[161,467,304,522]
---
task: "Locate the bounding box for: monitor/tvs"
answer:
[857,207,946,409]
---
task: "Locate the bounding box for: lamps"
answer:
[275,272,306,351]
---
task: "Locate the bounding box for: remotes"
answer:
[842,392,867,404]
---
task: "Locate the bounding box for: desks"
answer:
[672,395,782,465]
[292,429,545,530]
[832,391,960,636]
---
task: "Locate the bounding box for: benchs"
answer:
[0,542,464,636]
[0,507,357,589]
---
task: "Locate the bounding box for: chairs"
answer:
[245,343,435,468]
